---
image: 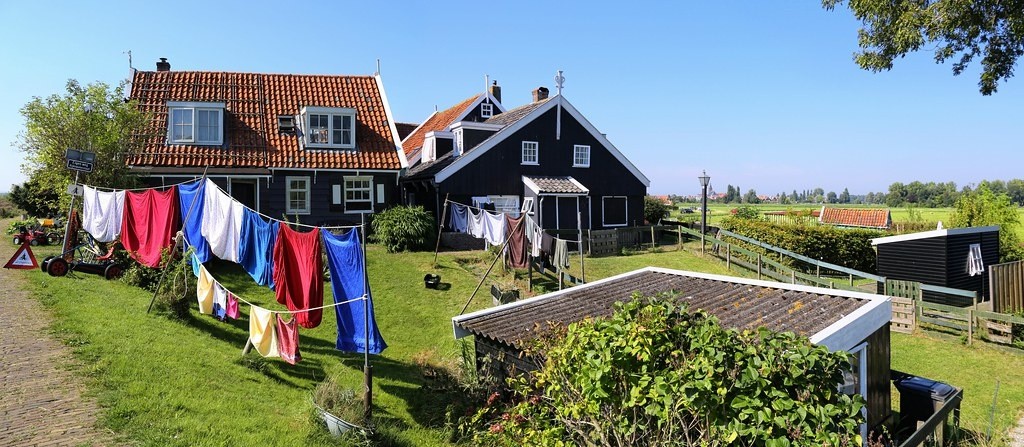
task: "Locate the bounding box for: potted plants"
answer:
[491,282,519,307]
[313,364,343,424]
[324,388,372,439]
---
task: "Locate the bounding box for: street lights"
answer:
[699,170,711,254]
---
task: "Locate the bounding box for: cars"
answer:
[680,208,693,213]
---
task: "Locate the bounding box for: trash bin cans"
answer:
[893,375,958,447]
